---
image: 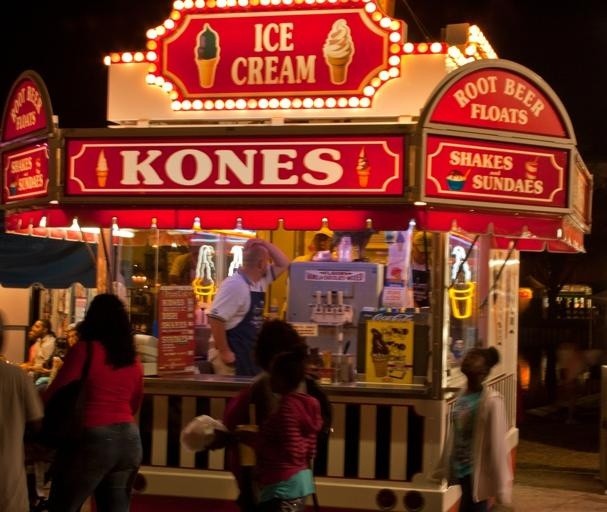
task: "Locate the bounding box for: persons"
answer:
[431,344,515,512]
[202,353,324,509]
[408,231,431,308]
[520,294,546,386]
[206,235,290,373]
[289,232,334,263]
[24,287,152,373]
[220,317,335,511]
[0,309,45,512]
[35,291,143,510]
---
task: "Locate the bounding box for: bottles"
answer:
[337,235,353,264]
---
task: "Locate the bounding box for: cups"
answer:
[320,251,331,262]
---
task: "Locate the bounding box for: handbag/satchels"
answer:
[31,337,92,448]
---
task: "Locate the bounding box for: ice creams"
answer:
[195,24,221,88]
[96,150,107,188]
[356,146,370,188]
[323,19,354,84]
[370,328,389,378]
